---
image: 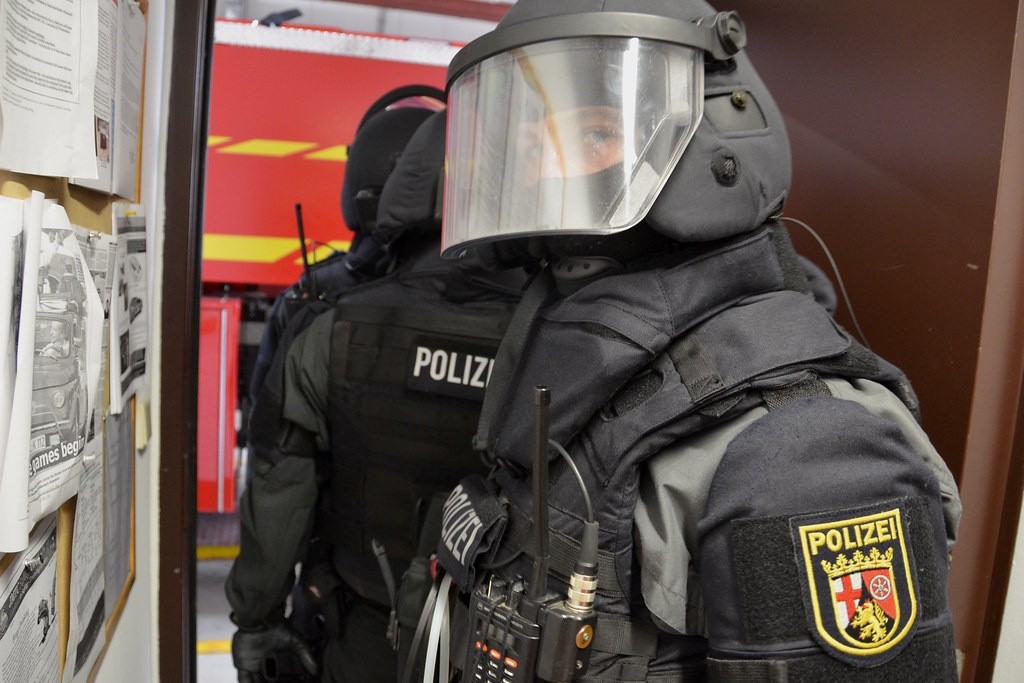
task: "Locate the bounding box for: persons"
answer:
[225,82,526,682]
[387,12,966,682]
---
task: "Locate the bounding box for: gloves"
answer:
[229,609,317,683]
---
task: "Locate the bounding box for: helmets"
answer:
[342,83,447,234]
[437,0,730,263]
